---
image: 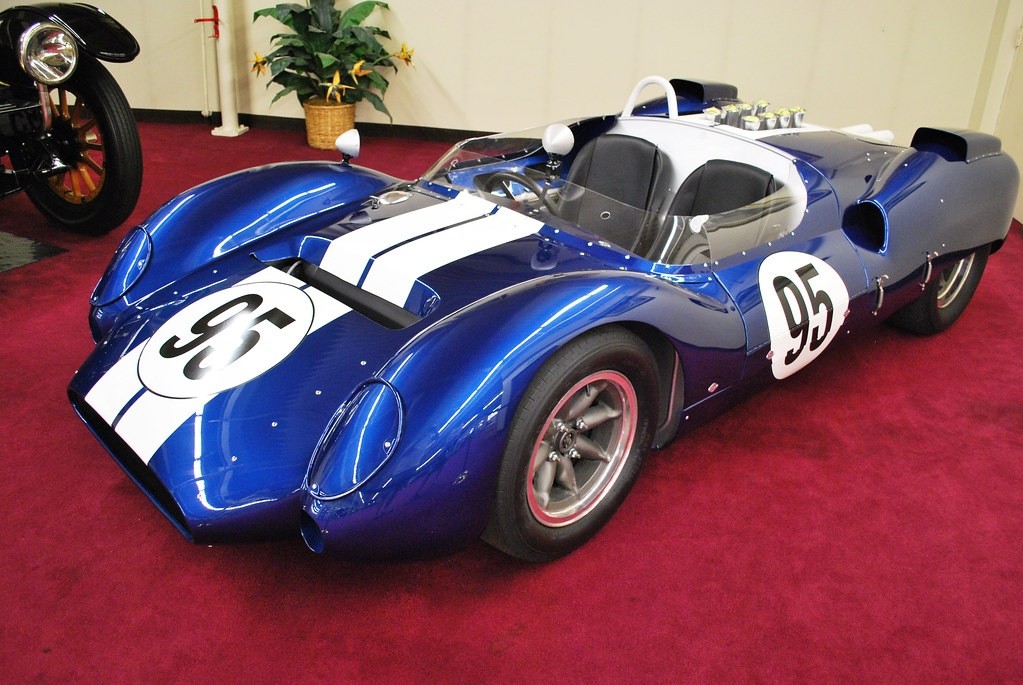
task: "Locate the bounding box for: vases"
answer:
[302,99,356,151]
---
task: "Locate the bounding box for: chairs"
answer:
[644,159,777,264]
[557,133,668,258]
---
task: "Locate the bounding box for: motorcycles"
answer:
[1,1,141,236]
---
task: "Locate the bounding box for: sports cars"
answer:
[64,77,1021,564]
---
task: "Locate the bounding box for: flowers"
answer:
[250,0,414,127]
